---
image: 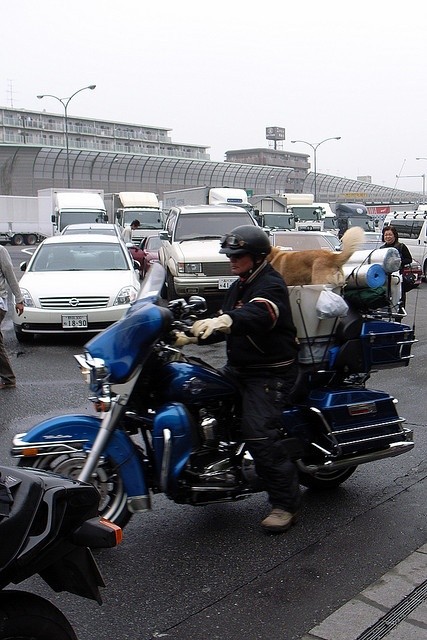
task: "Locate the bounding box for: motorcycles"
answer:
[0,463,123,640]
[10,260,420,531]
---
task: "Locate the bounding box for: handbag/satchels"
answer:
[398,243,416,292]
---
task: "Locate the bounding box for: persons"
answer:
[378,226,412,324]
[0,243,26,390]
[172,225,302,531]
[121,219,141,250]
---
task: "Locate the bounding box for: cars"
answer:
[130,234,162,279]
[318,232,341,250]
[9,232,142,343]
[268,228,336,253]
[337,239,423,286]
[59,223,122,236]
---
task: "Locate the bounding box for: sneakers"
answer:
[261,508,299,534]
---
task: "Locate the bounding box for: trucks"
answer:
[247,195,295,227]
[162,185,259,222]
[313,202,337,231]
[282,193,327,230]
[0,195,37,245]
[104,190,163,247]
[36,188,109,238]
[330,202,376,237]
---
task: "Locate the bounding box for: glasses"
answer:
[219,233,243,248]
[225,253,247,258]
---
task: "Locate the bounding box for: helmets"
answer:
[219,225,271,254]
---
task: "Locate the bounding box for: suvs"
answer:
[159,203,265,302]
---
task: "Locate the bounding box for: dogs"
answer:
[266,225,365,284]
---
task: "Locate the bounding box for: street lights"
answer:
[291,137,342,202]
[36,84,96,188]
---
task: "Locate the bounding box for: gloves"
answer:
[190,314,233,340]
